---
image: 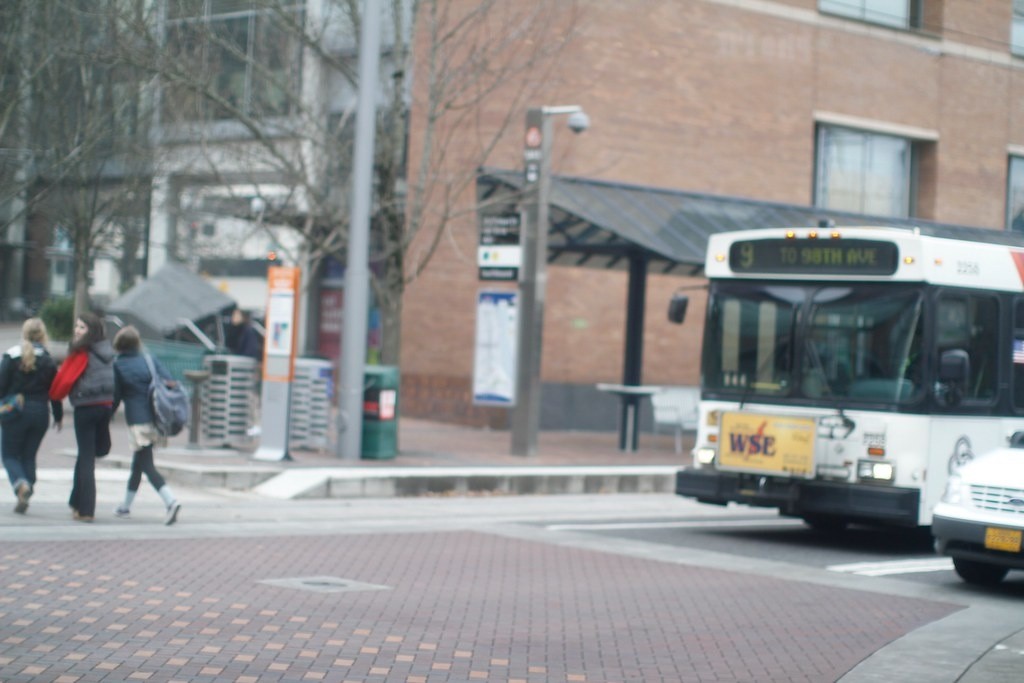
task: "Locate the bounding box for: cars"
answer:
[930,432,1024,585]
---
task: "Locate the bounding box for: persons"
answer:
[230,310,258,356]
[108,326,180,525]
[0,316,63,513]
[899,335,922,382]
[49,313,115,521]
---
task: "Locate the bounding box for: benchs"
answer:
[650,385,697,454]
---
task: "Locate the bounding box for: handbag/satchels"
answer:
[0,393,25,423]
[143,353,190,436]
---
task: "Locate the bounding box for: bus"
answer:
[674,225,1024,530]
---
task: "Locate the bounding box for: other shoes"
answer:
[112,508,131,519]
[73,511,95,523]
[14,482,32,513]
[163,499,181,526]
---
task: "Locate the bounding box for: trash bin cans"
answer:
[361,366,400,461]
